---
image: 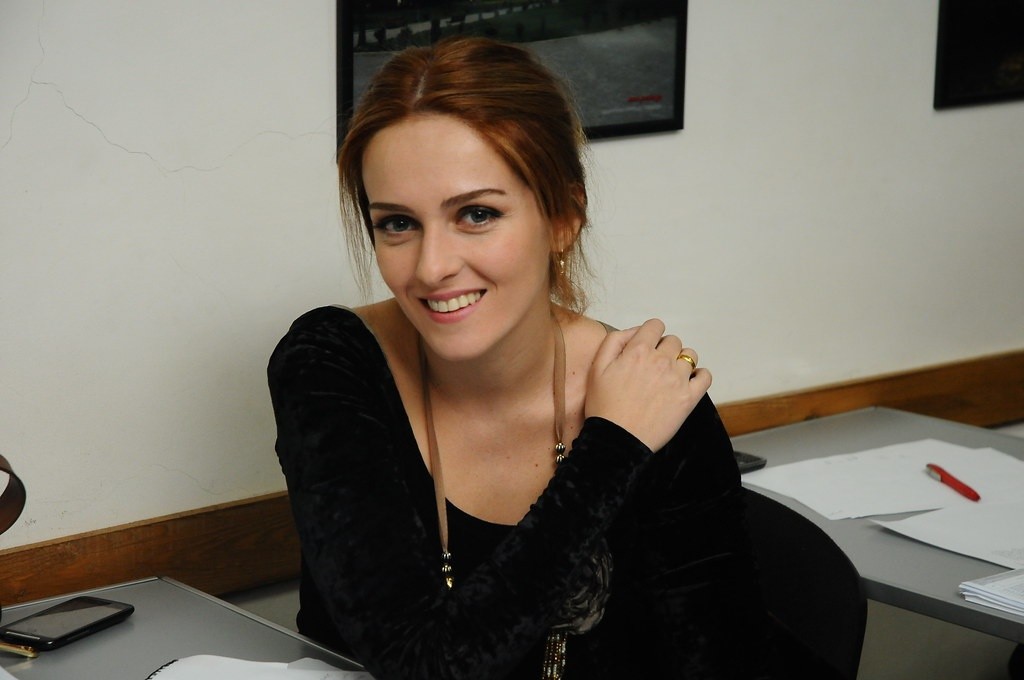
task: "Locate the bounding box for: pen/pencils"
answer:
[926,463,980,501]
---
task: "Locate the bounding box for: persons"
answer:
[266,37,748,680]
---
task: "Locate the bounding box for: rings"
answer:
[677,354,696,372]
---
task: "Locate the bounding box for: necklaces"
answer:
[416,311,565,590]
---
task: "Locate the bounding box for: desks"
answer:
[0,576,376,680]
[727,405,1024,644]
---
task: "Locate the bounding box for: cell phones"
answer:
[0,596,135,651]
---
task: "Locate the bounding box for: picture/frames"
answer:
[331,0,686,160]
